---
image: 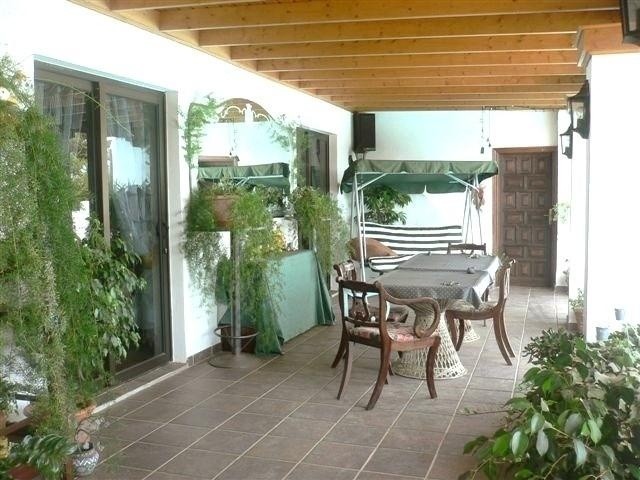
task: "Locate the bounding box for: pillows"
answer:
[346,237,395,260]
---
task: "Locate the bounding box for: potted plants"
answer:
[175,182,287,331]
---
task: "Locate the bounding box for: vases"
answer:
[71,442,99,476]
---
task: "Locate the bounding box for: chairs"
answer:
[335,275,441,410]
[447,241,486,255]
[330,260,409,366]
[445,258,517,366]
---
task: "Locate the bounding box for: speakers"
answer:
[353,113,376,153]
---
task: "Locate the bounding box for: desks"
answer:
[370,269,493,381]
[395,253,501,343]
[219,247,336,352]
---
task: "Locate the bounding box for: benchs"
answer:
[353,222,463,274]
